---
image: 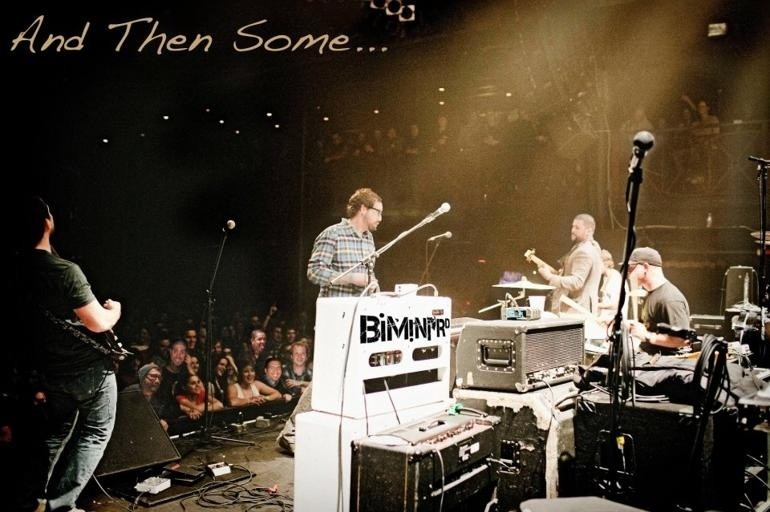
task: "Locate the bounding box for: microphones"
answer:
[428,230,453,242]
[747,154,770,166]
[222,220,235,233]
[427,202,452,220]
[627,130,654,174]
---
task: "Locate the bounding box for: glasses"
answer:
[146,374,163,381]
[364,204,382,215]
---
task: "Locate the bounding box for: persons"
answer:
[218,324,234,344]
[523,212,602,326]
[176,355,205,394]
[245,305,277,333]
[267,324,284,347]
[175,375,223,420]
[239,329,266,362]
[131,326,151,346]
[149,326,169,348]
[261,357,292,403]
[124,362,170,434]
[209,356,228,405]
[160,339,188,395]
[117,347,144,388]
[227,360,280,405]
[282,342,313,392]
[228,310,247,336]
[272,185,383,459]
[5,198,123,512]
[210,338,223,355]
[282,325,297,346]
[180,317,196,329]
[235,321,246,338]
[152,339,170,364]
[594,248,623,324]
[179,328,198,351]
[291,307,312,336]
[612,245,690,362]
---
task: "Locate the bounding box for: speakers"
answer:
[94,392,183,478]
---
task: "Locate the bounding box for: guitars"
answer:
[523,246,559,277]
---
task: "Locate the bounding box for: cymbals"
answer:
[490,274,557,291]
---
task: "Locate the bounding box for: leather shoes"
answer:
[279,438,295,454]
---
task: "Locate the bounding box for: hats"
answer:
[138,364,159,385]
[618,246,663,267]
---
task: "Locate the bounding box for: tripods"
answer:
[169,232,256,461]
